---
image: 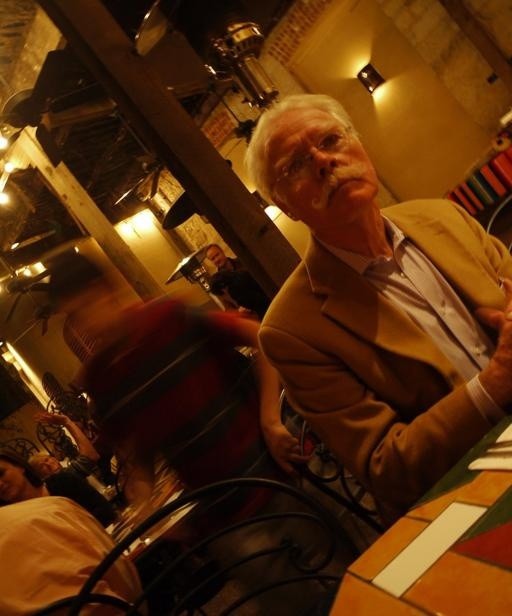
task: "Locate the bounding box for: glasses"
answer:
[277,126,353,182]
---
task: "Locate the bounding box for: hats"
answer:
[33,251,104,323]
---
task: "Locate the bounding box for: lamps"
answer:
[357,64,384,94]
[115,112,233,230]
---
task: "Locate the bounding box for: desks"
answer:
[332,410,511,616]
[437,133,512,218]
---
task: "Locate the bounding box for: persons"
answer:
[0,497,147,616]
[202,243,268,319]
[29,454,60,477]
[32,411,101,480]
[44,252,358,616]
[0,447,118,532]
[242,93,512,507]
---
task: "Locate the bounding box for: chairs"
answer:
[70,385,389,615]
